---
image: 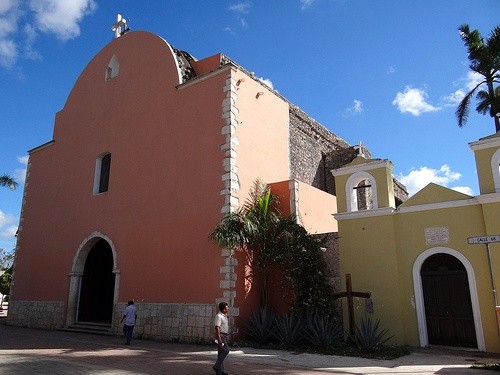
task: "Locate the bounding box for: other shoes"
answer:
[125,340,131,345]
[213,366,228,375]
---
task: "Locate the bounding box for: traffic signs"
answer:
[465,235,500,244]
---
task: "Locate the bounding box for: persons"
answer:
[120,300,138,345]
[212,301,229,375]
